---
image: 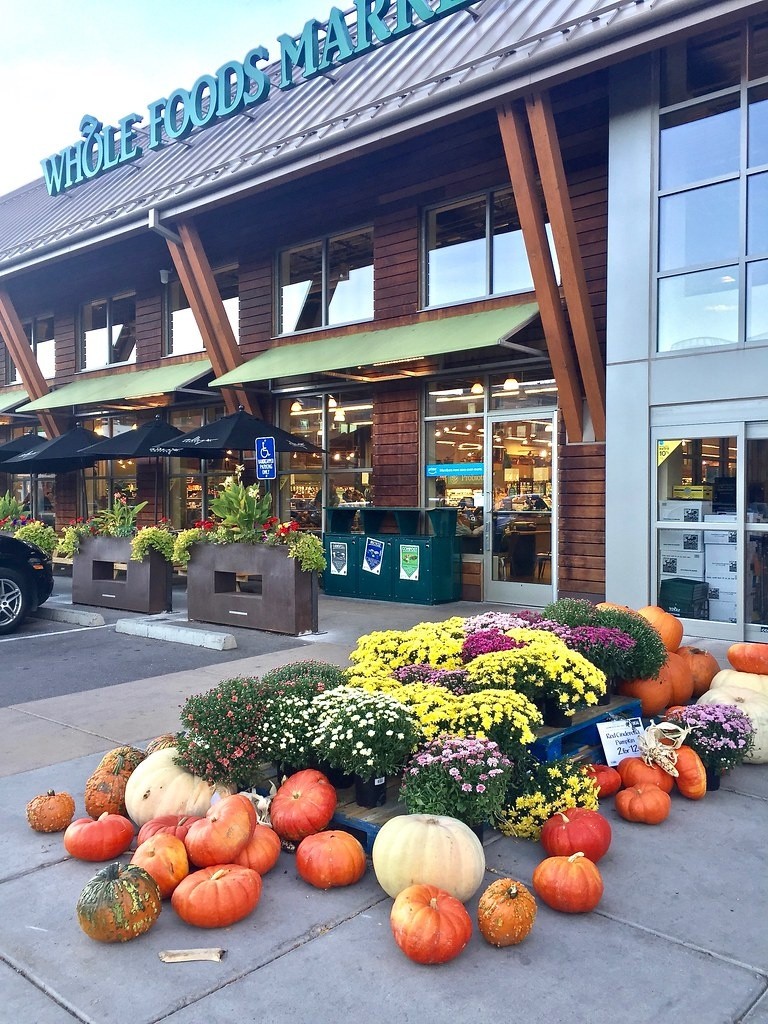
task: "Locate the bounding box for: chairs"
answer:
[536,523,552,578]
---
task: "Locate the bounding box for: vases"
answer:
[325,760,354,788]
[450,811,484,847]
[699,753,720,792]
[353,770,387,809]
[544,695,572,727]
[595,680,612,706]
[274,760,294,782]
[534,694,547,721]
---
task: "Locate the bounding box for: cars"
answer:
[0,532,54,636]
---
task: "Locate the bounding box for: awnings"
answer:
[207,300,550,399]
[0,389,41,419]
[13,359,224,422]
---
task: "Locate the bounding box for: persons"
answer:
[340,487,366,503]
[435,479,447,506]
[312,479,338,507]
[43,491,55,511]
[472,505,485,535]
[114,486,131,506]
[132,490,138,498]
[532,495,551,511]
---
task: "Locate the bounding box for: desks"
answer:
[506,529,550,577]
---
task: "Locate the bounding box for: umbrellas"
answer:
[77,413,239,526]
[152,400,330,483]
[0,422,114,524]
[0,429,49,520]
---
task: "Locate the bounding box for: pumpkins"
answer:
[25,602,768,966]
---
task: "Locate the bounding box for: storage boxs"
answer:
[657,475,765,621]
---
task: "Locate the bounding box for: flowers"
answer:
[57,490,175,564]
[0,489,58,551]
[171,598,763,842]
[171,465,328,572]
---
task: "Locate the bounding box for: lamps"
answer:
[471,376,483,393]
[327,395,346,421]
[503,372,519,390]
[291,397,302,412]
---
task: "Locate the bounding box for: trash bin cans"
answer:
[320,505,463,605]
